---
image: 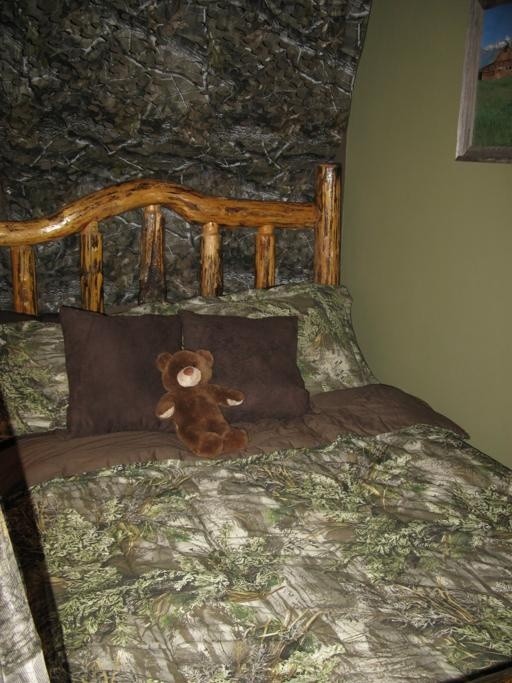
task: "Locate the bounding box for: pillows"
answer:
[181,308,309,424]
[181,283,376,396]
[58,303,178,431]
[0,301,179,436]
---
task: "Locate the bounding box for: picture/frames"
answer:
[454,1,511,163]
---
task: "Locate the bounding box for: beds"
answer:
[1,161,512,683]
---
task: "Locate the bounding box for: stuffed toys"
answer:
[157,350,247,457]
